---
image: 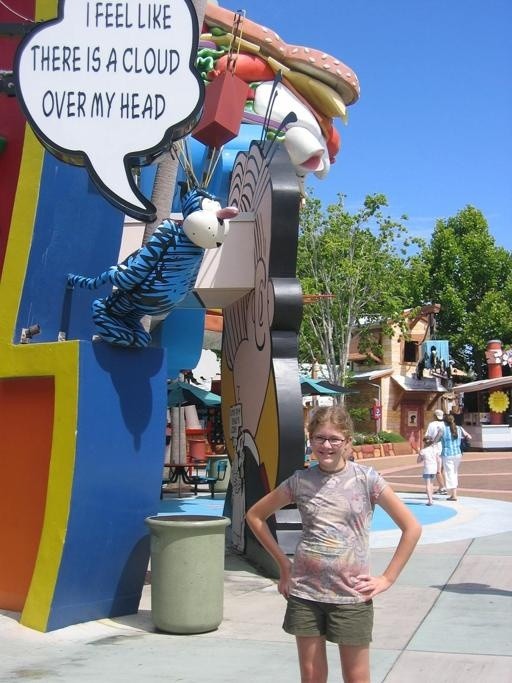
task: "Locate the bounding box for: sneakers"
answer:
[426,488,456,506]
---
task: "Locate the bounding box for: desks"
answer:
[161,462,208,498]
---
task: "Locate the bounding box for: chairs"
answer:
[205,460,228,500]
[192,458,211,492]
[161,476,171,499]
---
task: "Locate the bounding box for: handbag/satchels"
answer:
[461,436,471,449]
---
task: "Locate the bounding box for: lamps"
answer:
[19,323,40,343]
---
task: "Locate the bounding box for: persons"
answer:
[434,415,472,500]
[425,410,447,495]
[246,405,422,683]
[417,436,442,505]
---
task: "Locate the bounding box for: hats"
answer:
[435,410,443,420]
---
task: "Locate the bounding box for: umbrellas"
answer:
[300,376,361,395]
[167,381,222,463]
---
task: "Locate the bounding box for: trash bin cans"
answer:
[188,439,206,462]
[205,454,231,492]
[144,515,231,634]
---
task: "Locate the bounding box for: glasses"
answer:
[310,436,346,445]
